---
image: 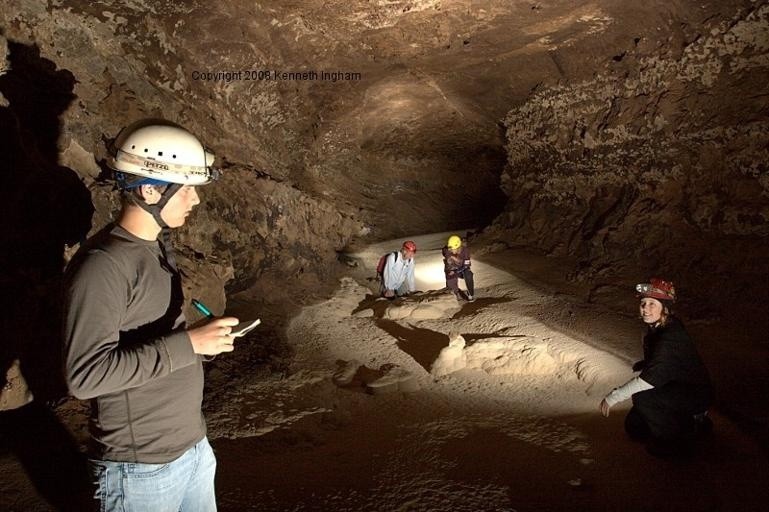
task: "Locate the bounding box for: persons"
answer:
[442,235,474,302]
[378,240,416,298]
[599,277,714,455]
[62,125,239,512]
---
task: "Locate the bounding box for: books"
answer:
[231,319,261,337]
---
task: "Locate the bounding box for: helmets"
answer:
[105,123,220,186]
[403,240,416,252]
[633,277,678,305]
[447,235,462,250]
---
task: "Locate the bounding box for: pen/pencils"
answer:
[191,298,217,319]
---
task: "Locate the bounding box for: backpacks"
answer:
[377,251,411,280]
